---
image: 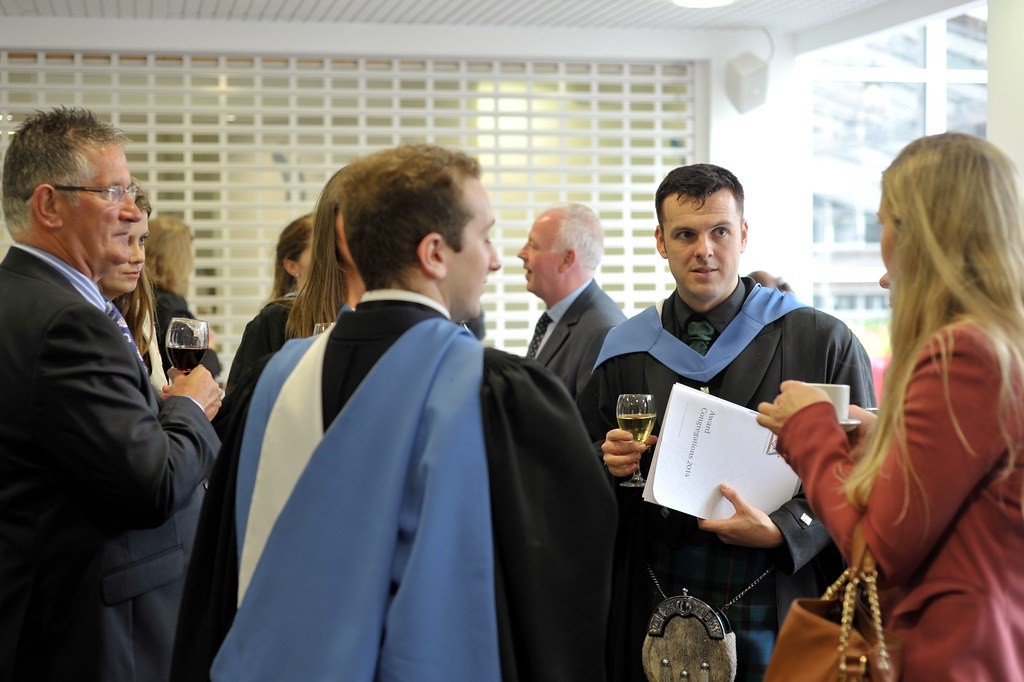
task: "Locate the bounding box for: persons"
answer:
[170,146,620,682]
[283,161,366,341]
[577,162,878,682]
[516,202,628,403]
[756,131,1023,682]
[748,270,794,297]
[95,184,173,399]
[220,211,317,396]
[0,104,223,682]
[111,214,224,379]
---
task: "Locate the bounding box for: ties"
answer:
[526,312,553,359]
[687,312,715,357]
[102,295,149,377]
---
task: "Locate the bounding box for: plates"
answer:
[838,419,861,431]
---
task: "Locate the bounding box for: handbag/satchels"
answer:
[641,588,736,682]
[763,520,904,682]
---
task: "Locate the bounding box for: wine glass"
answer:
[616,394,657,486]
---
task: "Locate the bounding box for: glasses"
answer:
[25,182,136,207]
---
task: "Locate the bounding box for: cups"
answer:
[165,317,209,375]
[314,323,332,335]
[808,383,850,420]
